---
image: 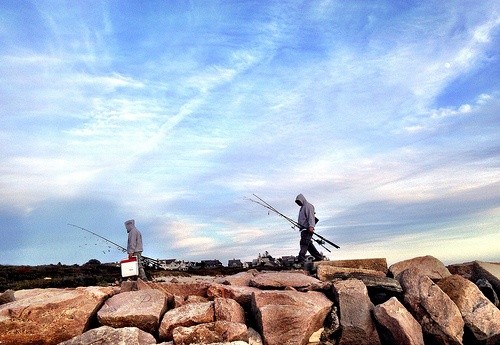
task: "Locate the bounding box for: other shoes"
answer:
[312,257,323,261]
[293,262,304,268]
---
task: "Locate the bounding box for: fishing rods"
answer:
[253,193,331,252]
[242,196,340,248]
[65,223,162,269]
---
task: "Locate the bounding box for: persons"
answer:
[293,194,322,264]
[118,220,147,284]
